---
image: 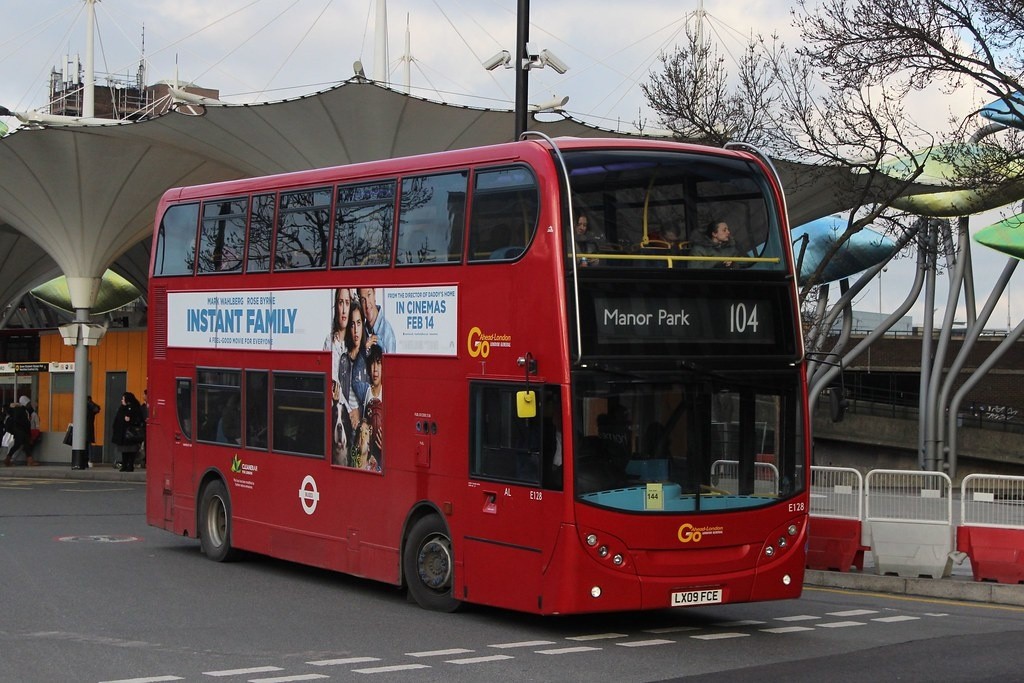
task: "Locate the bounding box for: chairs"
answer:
[294,249,320,268]
[215,416,229,443]
[639,239,673,268]
[552,429,563,466]
[630,243,640,268]
[676,241,689,268]
[211,254,240,271]
[599,244,624,267]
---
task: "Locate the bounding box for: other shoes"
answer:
[126,467,136,472]
[119,466,128,472]
[4,456,16,466]
[27,457,40,467]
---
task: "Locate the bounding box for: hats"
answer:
[19,396,30,406]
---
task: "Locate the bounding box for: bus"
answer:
[144,129,848,617]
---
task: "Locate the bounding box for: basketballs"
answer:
[365,397,383,450]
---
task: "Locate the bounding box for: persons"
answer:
[68,395,100,468]
[566,213,599,264]
[216,392,242,445]
[323,288,397,473]
[687,219,754,268]
[553,402,584,468]
[649,221,688,250]
[0,395,40,465]
[111,388,148,471]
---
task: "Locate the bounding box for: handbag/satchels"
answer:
[63,422,73,446]
[124,422,145,441]
